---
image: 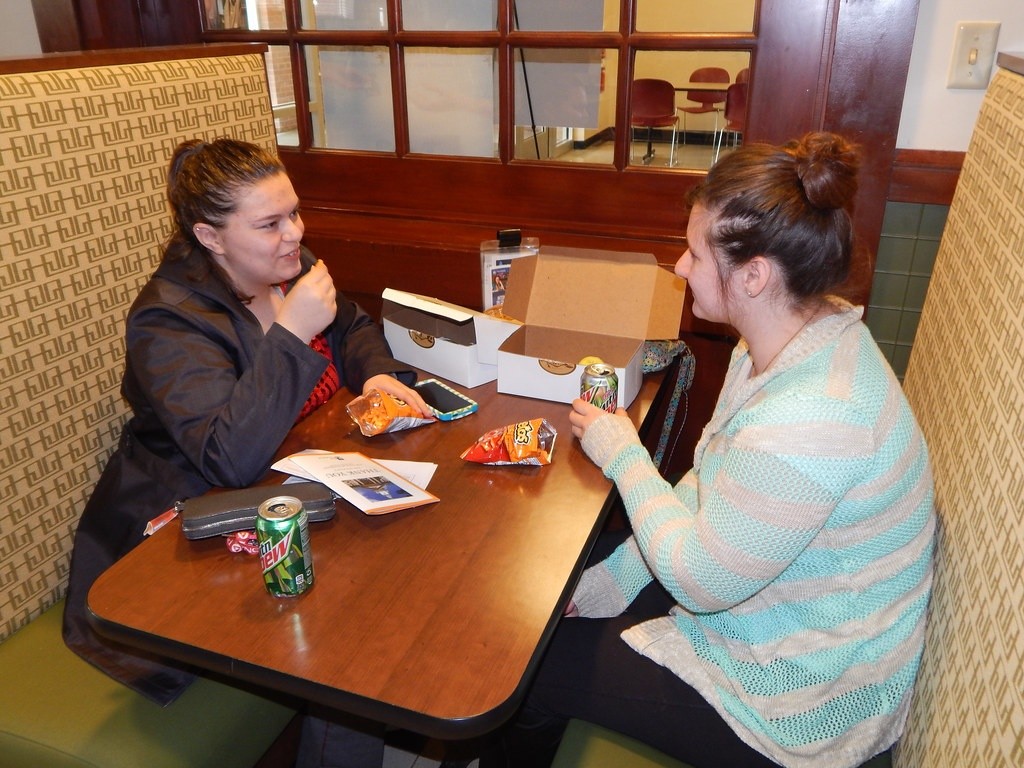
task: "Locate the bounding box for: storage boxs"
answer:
[498,243,689,414]
[381,288,521,388]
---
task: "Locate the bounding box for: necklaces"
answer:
[748,299,827,376]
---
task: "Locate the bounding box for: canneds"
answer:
[580,363,618,414]
[255,495,315,597]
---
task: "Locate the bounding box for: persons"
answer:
[62,140,433,768]
[479,132,935,768]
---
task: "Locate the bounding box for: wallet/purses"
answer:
[143,482,339,539]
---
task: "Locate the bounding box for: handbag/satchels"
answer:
[642,340,695,391]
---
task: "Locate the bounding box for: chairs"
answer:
[675,67,732,155]
[715,81,747,165]
[732,68,749,148]
[630,78,680,168]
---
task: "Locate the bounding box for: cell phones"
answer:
[410,378,478,421]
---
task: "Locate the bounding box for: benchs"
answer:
[551,52,1024,767]
[0,40,332,768]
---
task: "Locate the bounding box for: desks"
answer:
[643,82,732,165]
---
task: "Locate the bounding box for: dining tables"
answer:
[84,322,669,768]
